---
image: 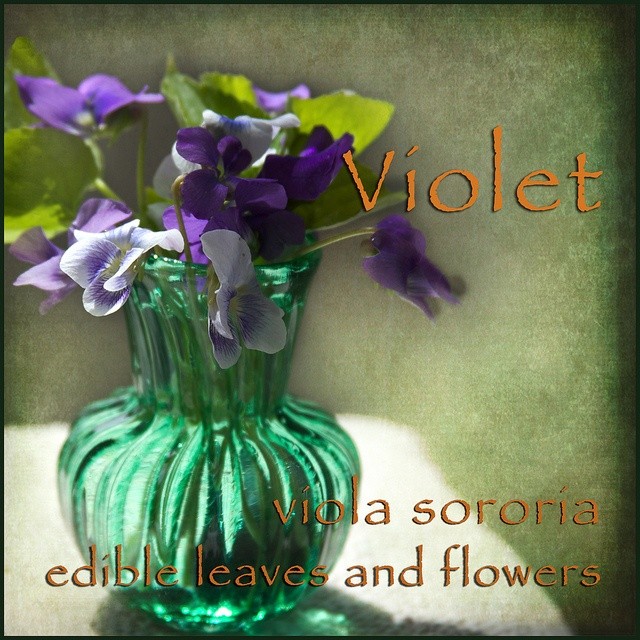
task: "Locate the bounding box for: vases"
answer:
[57,253,361,637]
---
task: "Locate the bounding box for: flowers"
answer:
[9,76,458,369]
